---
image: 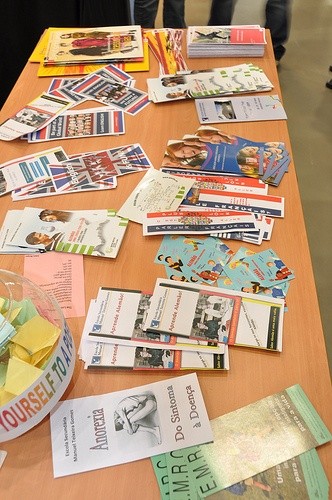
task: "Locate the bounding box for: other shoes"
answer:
[326,79,332,89]
[274,45,286,65]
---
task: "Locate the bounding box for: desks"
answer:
[1,27,332,499]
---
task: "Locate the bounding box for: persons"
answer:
[135,349,164,367]
[167,142,208,167]
[134,0,187,29]
[135,319,160,339]
[25,232,65,251]
[39,208,72,223]
[166,89,193,99]
[196,130,233,144]
[207,0,290,67]
[57,30,138,56]
[161,76,185,87]
[192,320,220,339]
